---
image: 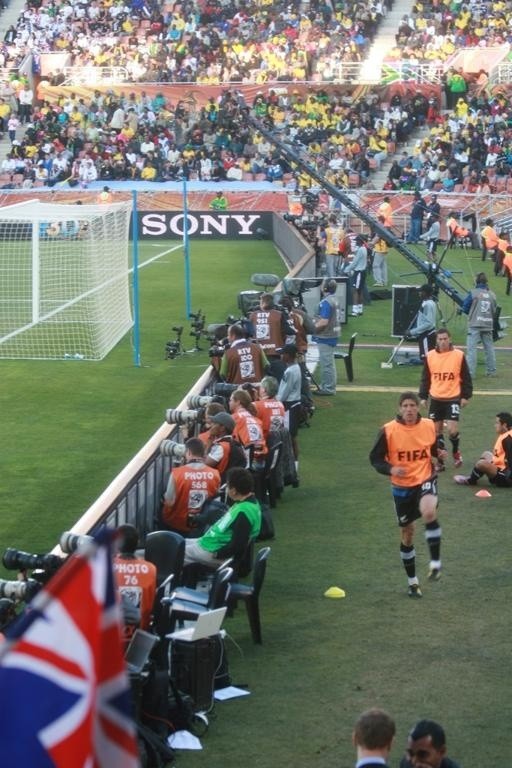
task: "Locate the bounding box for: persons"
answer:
[3,1,512,768]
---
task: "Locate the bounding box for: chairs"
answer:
[42,0,191,53]
[228,546,270,645]
[168,583,232,633]
[505,265,512,295]
[77,141,94,162]
[260,32,330,81]
[264,441,283,508]
[170,566,234,629]
[449,226,468,250]
[211,555,235,592]
[433,169,512,194]
[481,236,498,262]
[145,531,186,593]
[0,173,45,189]
[237,156,376,189]
[334,331,358,381]
[494,246,505,278]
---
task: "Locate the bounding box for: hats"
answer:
[207,411,236,435]
[275,344,298,354]
[416,284,433,292]
[352,234,366,240]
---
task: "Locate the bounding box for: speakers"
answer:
[170,642,216,714]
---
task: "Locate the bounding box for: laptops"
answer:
[166,606,230,641]
[124,628,156,677]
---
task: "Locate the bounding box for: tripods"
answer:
[381,283,449,370]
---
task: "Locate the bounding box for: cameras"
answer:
[283,212,302,221]
[159,438,186,467]
[0,546,65,582]
[215,382,255,402]
[296,224,316,232]
[61,530,95,554]
[186,394,223,409]
[0,577,45,602]
[165,409,205,426]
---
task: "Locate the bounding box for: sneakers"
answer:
[455,474,477,485]
[453,450,463,466]
[427,568,443,582]
[313,389,333,395]
[407,584,423,598]
[434,461,446,472]
